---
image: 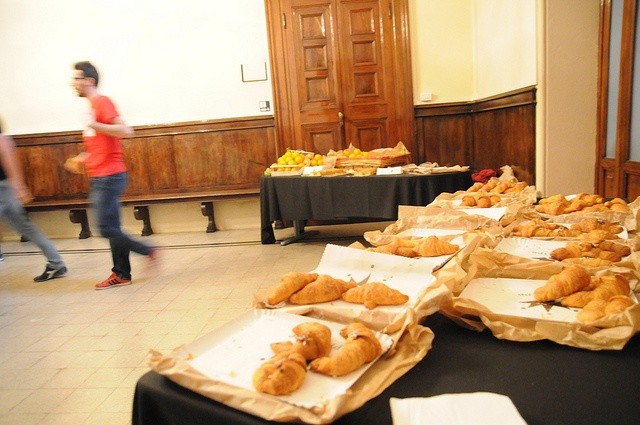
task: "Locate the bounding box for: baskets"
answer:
[336,154,412,174]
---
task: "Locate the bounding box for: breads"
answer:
[504,219,626,238]
[367,235,462,257]
[289,275,356,303]
[536,193,628,215]
[551,238,631,261]
[467,179,527,195]
[533,268,635,324]
[311,323,381,376]
[252,344,308,398]
[267,272,319,304]
[292,323,332,360]
[341,282,410,308]
[458,194,503,207]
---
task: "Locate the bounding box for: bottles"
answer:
[81,108,96,137]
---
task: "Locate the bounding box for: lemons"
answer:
[263,150,326,176]
[336,148,371,162]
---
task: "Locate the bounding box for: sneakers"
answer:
[94,273,132,289]
[33,264,68,282]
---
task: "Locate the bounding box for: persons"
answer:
[64,61,164,290]
[0,116,68,283]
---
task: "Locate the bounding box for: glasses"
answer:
[71,76,87,80]
